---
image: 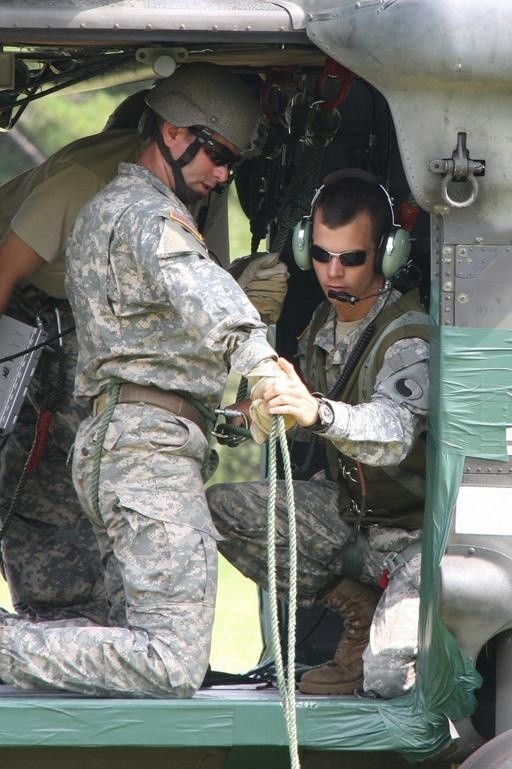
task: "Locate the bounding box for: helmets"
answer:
[144,62,261,150]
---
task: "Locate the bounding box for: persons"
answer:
[202,167,431,697]
[2,125,231,622]
[0,58,298,701]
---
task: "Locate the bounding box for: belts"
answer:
[91,383,208,431]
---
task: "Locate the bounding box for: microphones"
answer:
[328,279,392,305]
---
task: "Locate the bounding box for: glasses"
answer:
[310,243,377,269]
[185,127,243,171]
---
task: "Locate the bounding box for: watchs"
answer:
[301,390,336,433]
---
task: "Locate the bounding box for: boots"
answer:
[298,576,381,695]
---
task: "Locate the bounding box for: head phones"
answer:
[291,169,412,279]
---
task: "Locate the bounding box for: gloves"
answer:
[225,252,287,327]
[246,362,297,445]
